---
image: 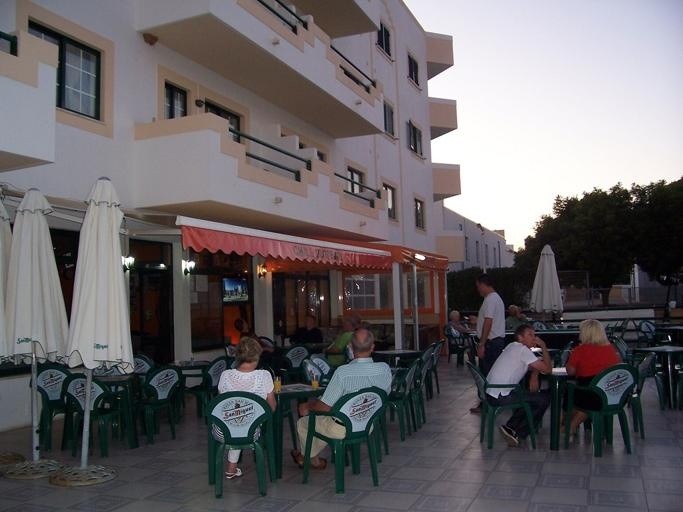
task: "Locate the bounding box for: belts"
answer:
[331,417,345,426]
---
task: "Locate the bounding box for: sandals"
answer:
[291,449,305,468]
[225,468,242,479]
[311,456,327,469]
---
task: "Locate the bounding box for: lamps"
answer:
[181,258,196,276]
[256,263,268,279]
[121,254,136,273]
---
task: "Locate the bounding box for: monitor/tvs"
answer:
[220,276,249,304]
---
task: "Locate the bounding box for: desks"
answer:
[374,349,422,422]
[272,384,325,476]
[634,344,683,410]
[542,365,584,451]
[655,325,683,333]
[469,331,581,368]
[94,374,140,449]
[171,361,211,419]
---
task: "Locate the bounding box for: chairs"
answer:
[564,364,638,457]
[301,386,387,494]
[326,338,354,380]
[61,373,125,458]
[131,365,183,445]
[627,352,656,438]
[305,341,331,354]
[444,326,476,368]
[554,348,569,367]
[421,338,447,399]
[393,342,436,429]
[604,321,619,338]
[185,356,229,423]
[258,336,275,348]
[29,363,80,452]
[610,337,664,410]
[106,355,152,434]
[617,336,662,394]
[206,393,278,499]
[533,320,548,330]
[614,318,630,338]
[225,342,237,368]
[563,287,590,310]
[639,320,657,346]
[284,345,309,383]
[464,322,478,347]
[385,358,418,441]
[464,359,537,450]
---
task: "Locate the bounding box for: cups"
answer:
[274,375,282,390]
[312,375,318,388]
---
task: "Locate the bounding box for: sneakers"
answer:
[499,424,520,448]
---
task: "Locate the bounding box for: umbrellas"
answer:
[0,187,70,481]
[47,175,135,488]
[529,244,563,321]
[0,184,27,479]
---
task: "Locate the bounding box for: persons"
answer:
[469,273,506,413]
[506,304,529,331]
[484,324,553,448]
[290,327,393,471]
[210,336,278,480]
[324,310,363,365]
[560,318,623,435]
[290,313,324,355]
[234,317,282,361]
[448,310,476,365]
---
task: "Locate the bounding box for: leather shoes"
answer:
[469,408,481,413]
